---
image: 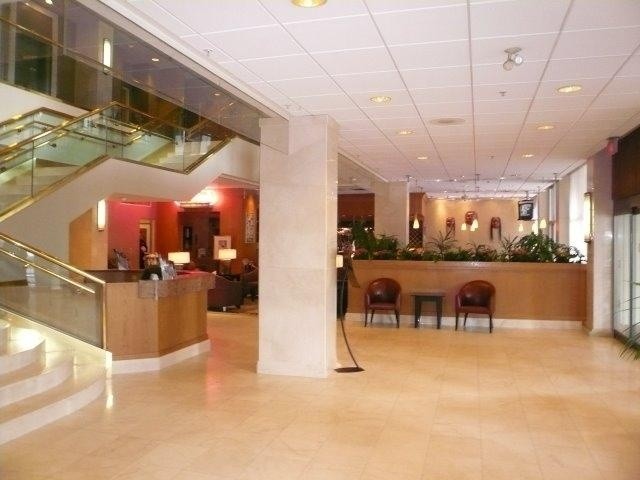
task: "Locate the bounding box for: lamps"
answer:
[98,200,106,232]
[103,37,112,73]
[460,171,480,233]
[168,252,192,274]
[502,47,524,72]
[218,249,237,276]
[412,175,420,229]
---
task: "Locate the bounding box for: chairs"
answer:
[365,277,402,328]
[205,259,259,313]
[454,278,495,334]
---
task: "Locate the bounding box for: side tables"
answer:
[409,292,445,330]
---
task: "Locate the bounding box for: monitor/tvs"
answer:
[518,203,533,220]
[141,265,162,280]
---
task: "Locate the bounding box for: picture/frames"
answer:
[214,235,232,260]
